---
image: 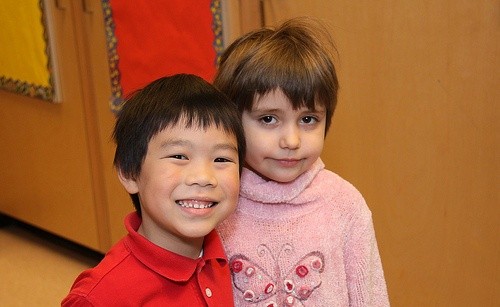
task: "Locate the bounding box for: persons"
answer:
[60,72,246,307]
[212,16,390,306]
